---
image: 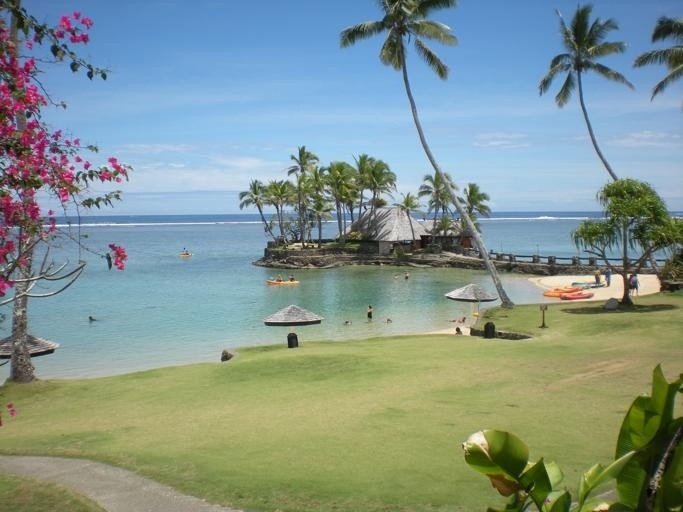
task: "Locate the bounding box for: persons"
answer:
[366,305,373,320]
[629,274,633,296]
[454,327,463,334]
[603,267,610,287]
[288,274,294,282]
[632,272,639,296]
[276,273,281,282]
[594,267,600,287]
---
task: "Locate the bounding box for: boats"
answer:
[180,253,191,256]
[266,280,298,284]
[544,281,605,300]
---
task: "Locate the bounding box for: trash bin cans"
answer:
[288,333,299,349]
[485,323,495,338]
